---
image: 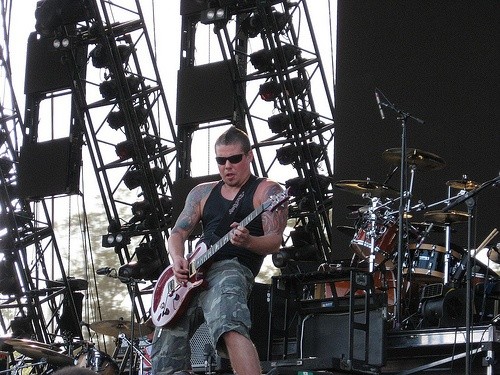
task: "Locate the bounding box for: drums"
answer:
[72,348,120,375]
[349,215,410,270]
[402,242,463,285]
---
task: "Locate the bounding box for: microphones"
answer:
[375,91,386,120]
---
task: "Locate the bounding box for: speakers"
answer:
[297,293,388,367]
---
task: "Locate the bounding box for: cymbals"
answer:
[334,179,401,197]
[378,146,446,173]
[423,209,474,226]
[445,175,481,189]
[3,337,75,367]
[337,224,358,235]
[90,316,153,338]
[346,203,367,210]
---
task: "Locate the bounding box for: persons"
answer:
[150,129,289,375]
[464,243,500,276]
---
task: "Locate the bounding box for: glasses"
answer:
[216,154,247,165]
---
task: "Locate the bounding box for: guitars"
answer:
[150,186,291,330]
